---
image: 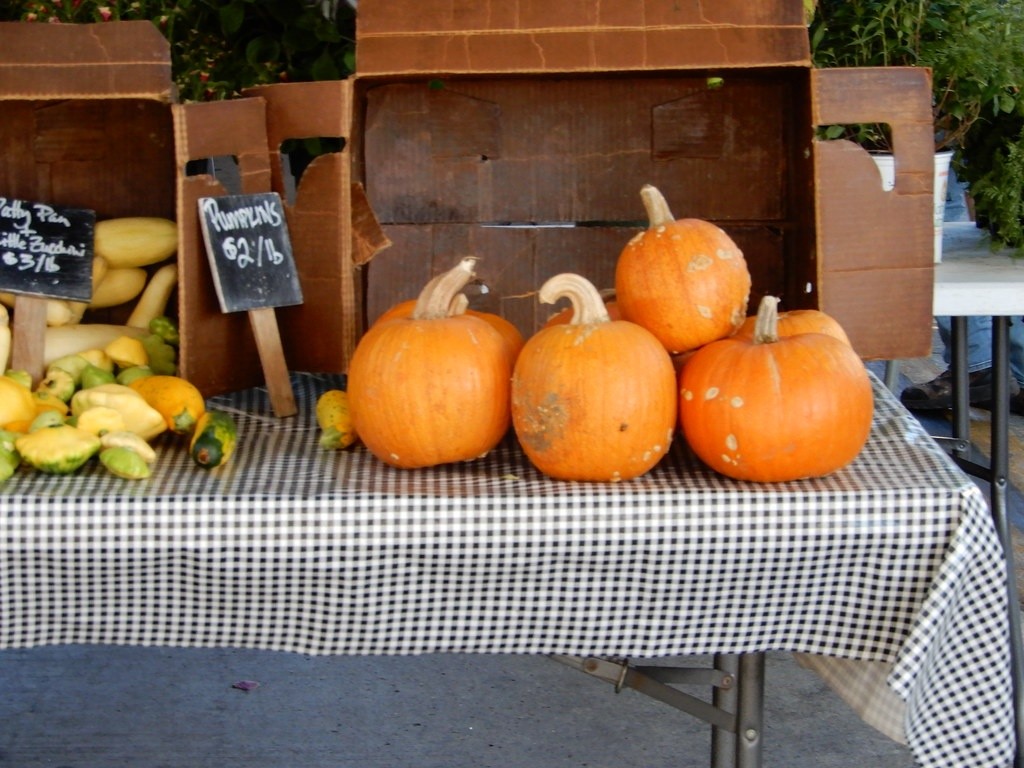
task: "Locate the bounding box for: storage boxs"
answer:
[0,0,937,401]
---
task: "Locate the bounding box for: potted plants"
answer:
[807,0,1024,263]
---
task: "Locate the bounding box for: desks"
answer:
[0,221,1024,768]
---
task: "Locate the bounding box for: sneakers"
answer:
[900,366,1019,409]
[969,386,1024,417]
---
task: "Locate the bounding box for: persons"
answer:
[899,128,1024,418]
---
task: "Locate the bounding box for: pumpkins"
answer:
[346,182,873,482]
[1,216,178,377]
